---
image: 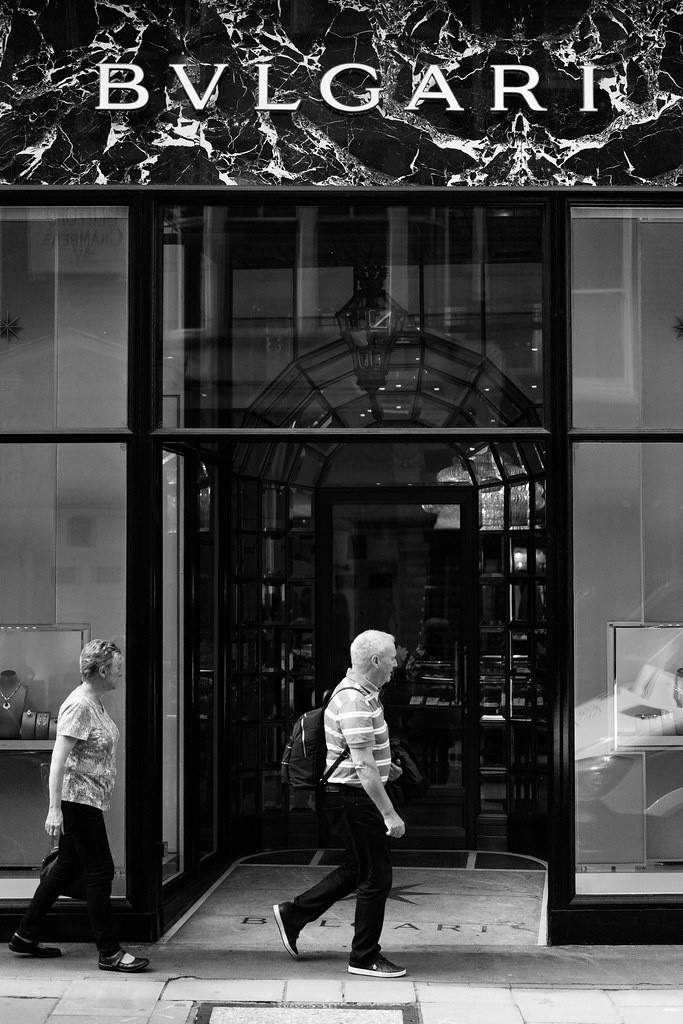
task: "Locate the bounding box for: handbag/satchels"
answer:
[39,834,77,897]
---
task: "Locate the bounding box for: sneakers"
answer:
[272,901,308,959]
[347,952,407,978]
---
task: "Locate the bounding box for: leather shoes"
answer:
[8,934,62,958]
[98,950,150,972]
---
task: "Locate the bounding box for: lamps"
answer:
[337,261,407,423]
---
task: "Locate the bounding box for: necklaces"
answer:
[1,681,22,710]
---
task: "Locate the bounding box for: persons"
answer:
[9,641,149,972]
[272,630,406,978]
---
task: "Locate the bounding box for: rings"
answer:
[27,710,31,716]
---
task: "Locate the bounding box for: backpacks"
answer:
[279,687,375,790]
